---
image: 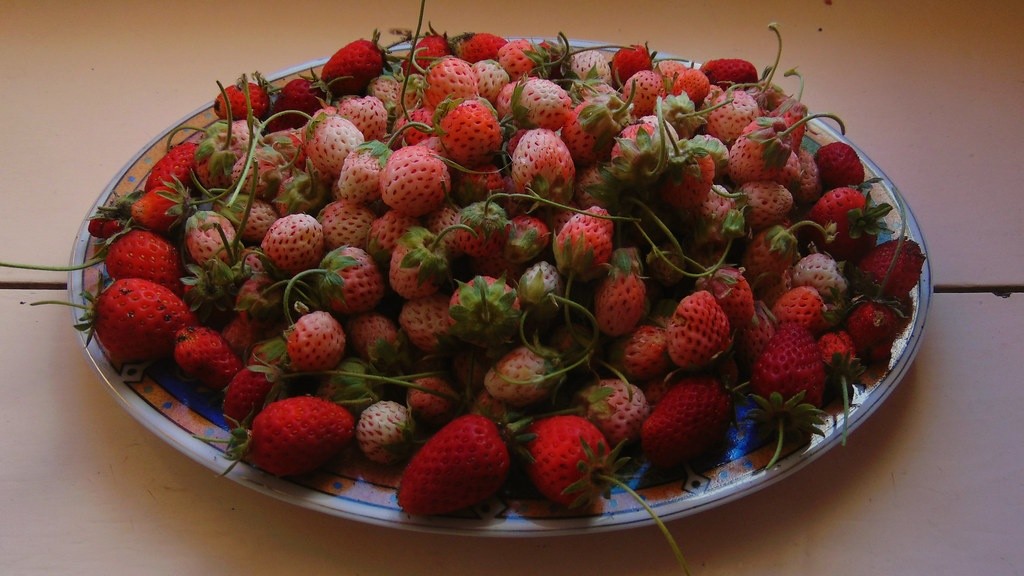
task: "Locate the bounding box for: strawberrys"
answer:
[71,21,926,521]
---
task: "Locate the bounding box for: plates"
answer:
[68,39,931,538]
